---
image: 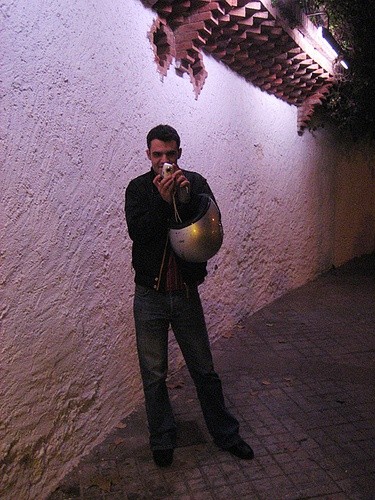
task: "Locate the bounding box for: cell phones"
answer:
[161,163,174,178]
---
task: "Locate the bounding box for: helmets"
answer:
[169,193,224,263]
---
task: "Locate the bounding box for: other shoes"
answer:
[215,436,255,460]
[152,433,176,466]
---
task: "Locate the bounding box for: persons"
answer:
[123,123,256,468]
